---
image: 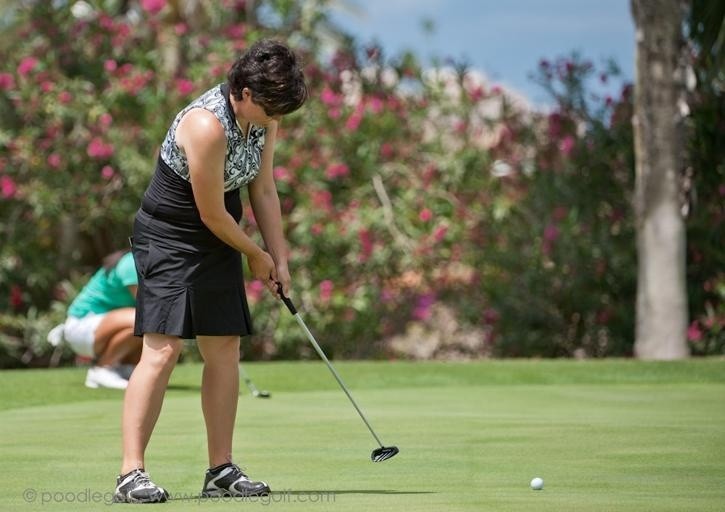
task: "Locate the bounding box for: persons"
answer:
[112,38,308,503]
[63,247,143,390]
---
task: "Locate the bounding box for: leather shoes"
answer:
[85,367,128,390]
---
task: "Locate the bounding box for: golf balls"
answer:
[531,477,545,489]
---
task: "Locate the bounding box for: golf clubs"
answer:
[271,278,398,462]
[238,365,271,397]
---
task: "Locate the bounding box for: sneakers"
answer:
[113,467,168,503]
[202,462,271,497]
